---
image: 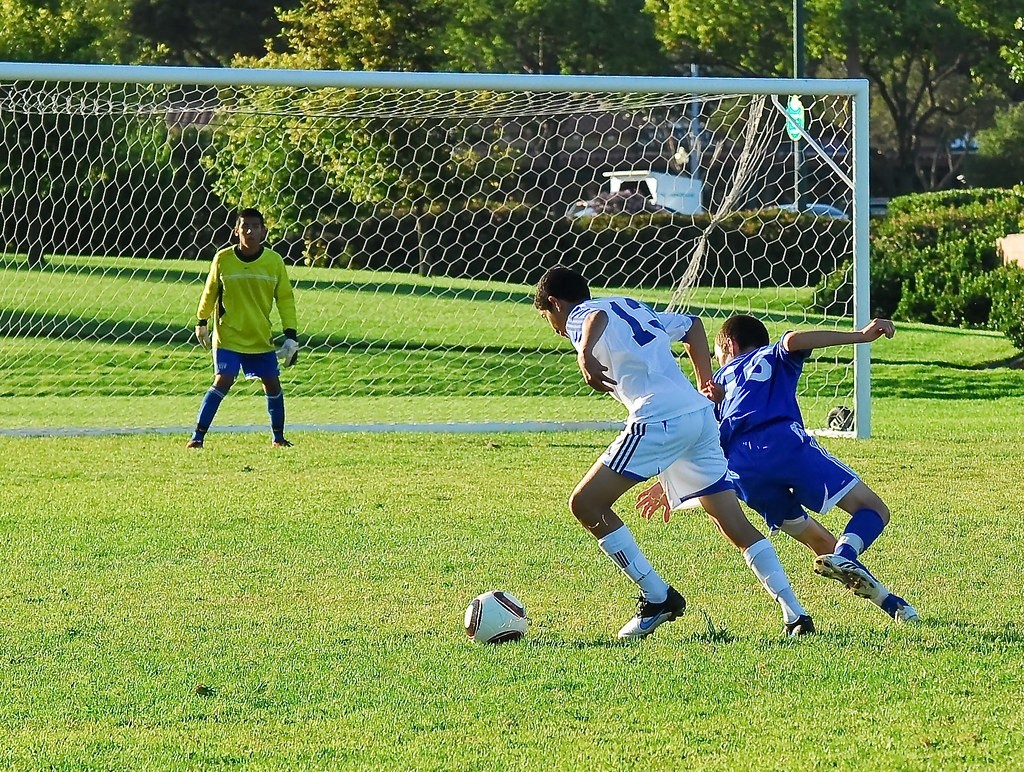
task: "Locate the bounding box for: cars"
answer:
[767,199,888,221]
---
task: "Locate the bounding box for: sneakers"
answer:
[814,554,881,600]
[783,615,815,636]
[618,585,686,638]
[894,602,919,625]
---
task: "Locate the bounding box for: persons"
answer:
[534,267,815,639]
[185,208,299,448]
[635,315,918,623]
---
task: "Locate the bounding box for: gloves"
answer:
[279,338,298,368]
[195,325,211,350]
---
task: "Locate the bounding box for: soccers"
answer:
[463,591,533,646]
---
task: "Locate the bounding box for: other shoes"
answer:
[186,440,203,448]
[273,439,294,448]
